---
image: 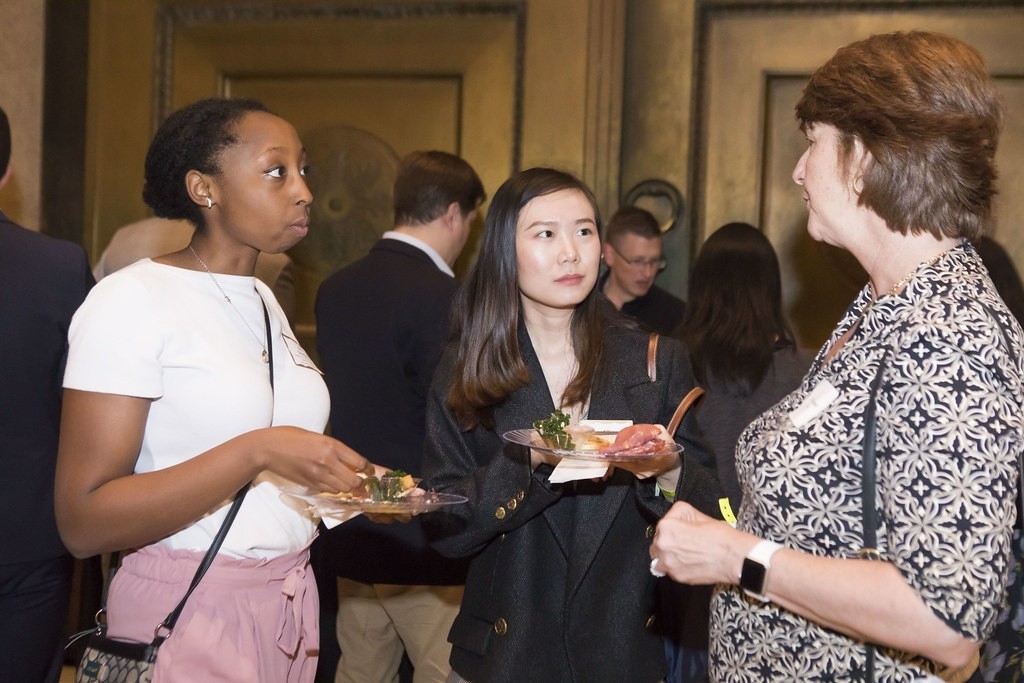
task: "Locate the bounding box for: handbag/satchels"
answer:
[74,635,158,683]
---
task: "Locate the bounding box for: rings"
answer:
[650,559,666,577]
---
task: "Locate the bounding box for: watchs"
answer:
[739,538,785,603]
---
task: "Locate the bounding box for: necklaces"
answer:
[188,246,270,364]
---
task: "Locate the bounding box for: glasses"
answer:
[612,245,667,272]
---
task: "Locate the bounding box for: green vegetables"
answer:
[362,468,415,502]
[533,409,576,450]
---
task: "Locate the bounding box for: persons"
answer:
[598,205,684,337]
[315,153,487,683]
[419,168,726,683]
[667,220,815,683]
[649,31,1024,683]
[54,97,422,683]
[92,210,296,336]
[0,110,97,683]
